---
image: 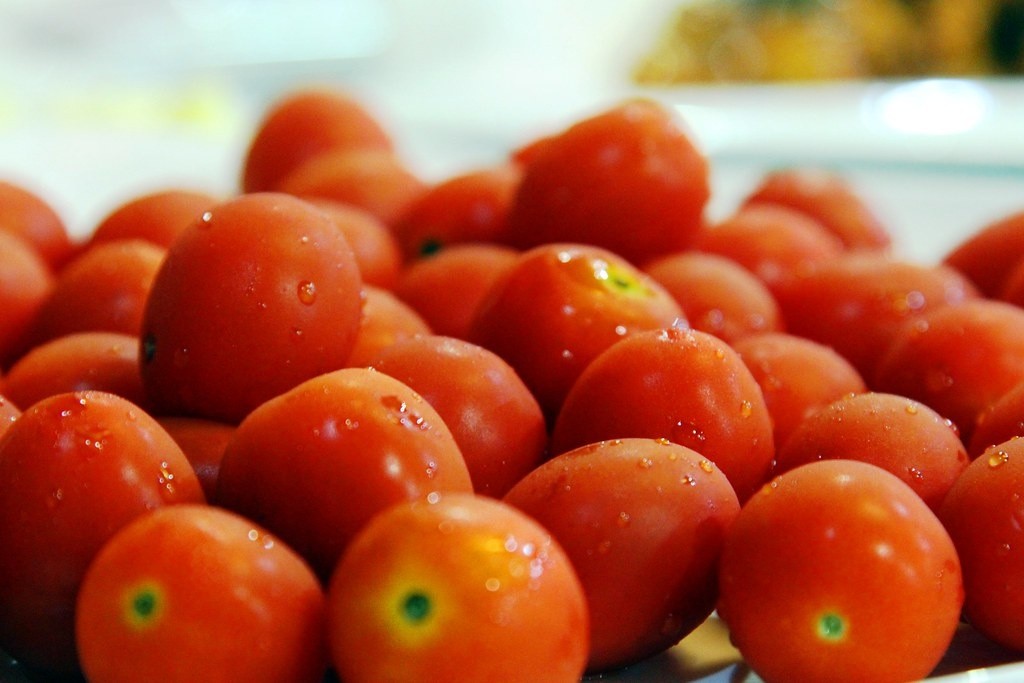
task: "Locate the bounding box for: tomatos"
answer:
[2,93,1023,683]
